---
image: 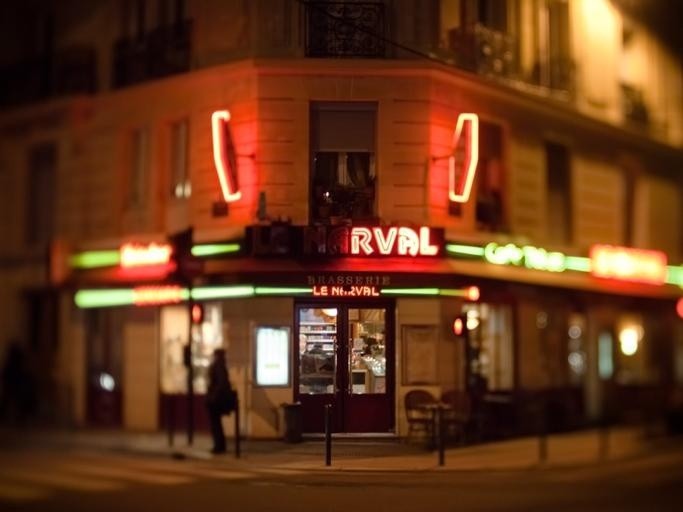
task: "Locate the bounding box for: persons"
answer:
[207,348,236,453]
[299,334,310,360]
